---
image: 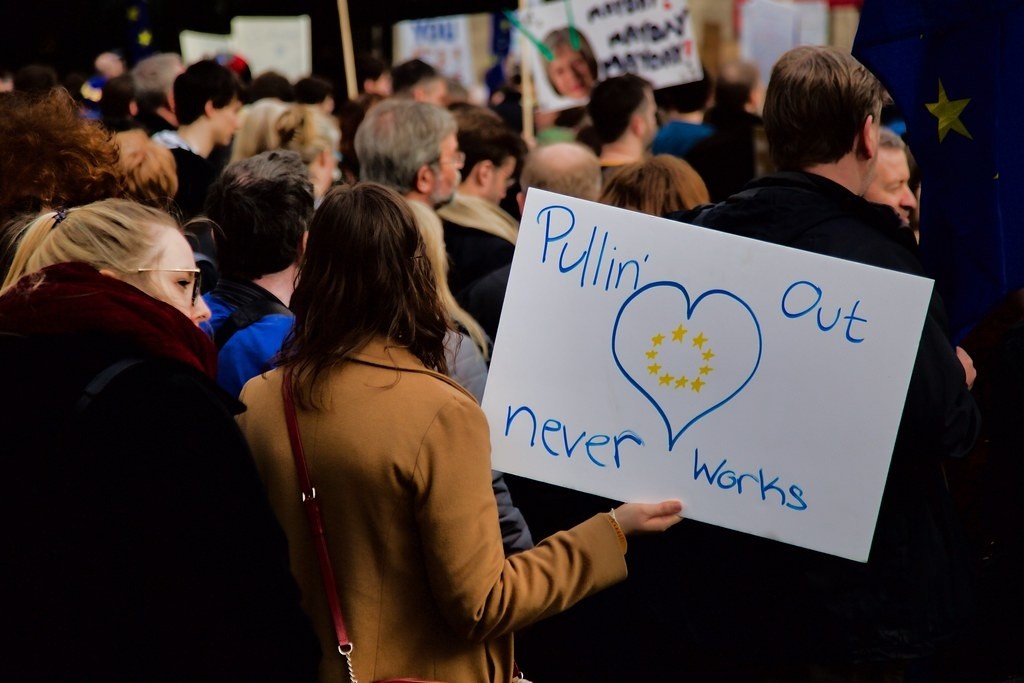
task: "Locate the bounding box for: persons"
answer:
[649,65,712,159]
[588,75,659,159]
[0,197,323,683]
[455,108,508,150]
[402,126,535,558]
[864,128,917,230]
[599,153,710,216]
[235,184,683,683]
[691,45,978,683]
[460,140,602,345]
[354,96,466,209]
[198,150,313,397]
[0,52,469,263]
[542,29,598,97]
[702,62,763,203]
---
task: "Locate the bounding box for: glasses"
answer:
[428,152,466,169]
[494,167,515,188]
[128,267,202,307]
